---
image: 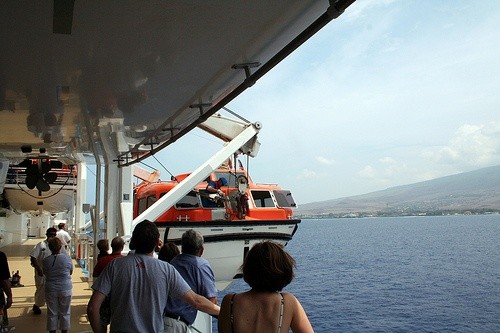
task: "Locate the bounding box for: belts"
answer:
[164,311,190,326]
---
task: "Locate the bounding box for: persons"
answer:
[163,230,218,333]
[41,237,74,333]
[86,219,222,333]
[57,222,71,256]
[203,177,228,207]
[0,250,13,333]
[218,240,314,333]
[31,228,57,314]
[159,241,181,264]
[97,238,109,261]
[92,237,125,277]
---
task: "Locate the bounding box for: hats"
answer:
[220,178,227,186]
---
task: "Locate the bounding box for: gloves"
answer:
[217,189,224,195]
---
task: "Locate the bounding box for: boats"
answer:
[112,160,301,290]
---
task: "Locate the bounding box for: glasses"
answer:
[48,234,56,237]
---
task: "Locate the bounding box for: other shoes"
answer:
[1,325,16,332]
[33,304,41,314]
